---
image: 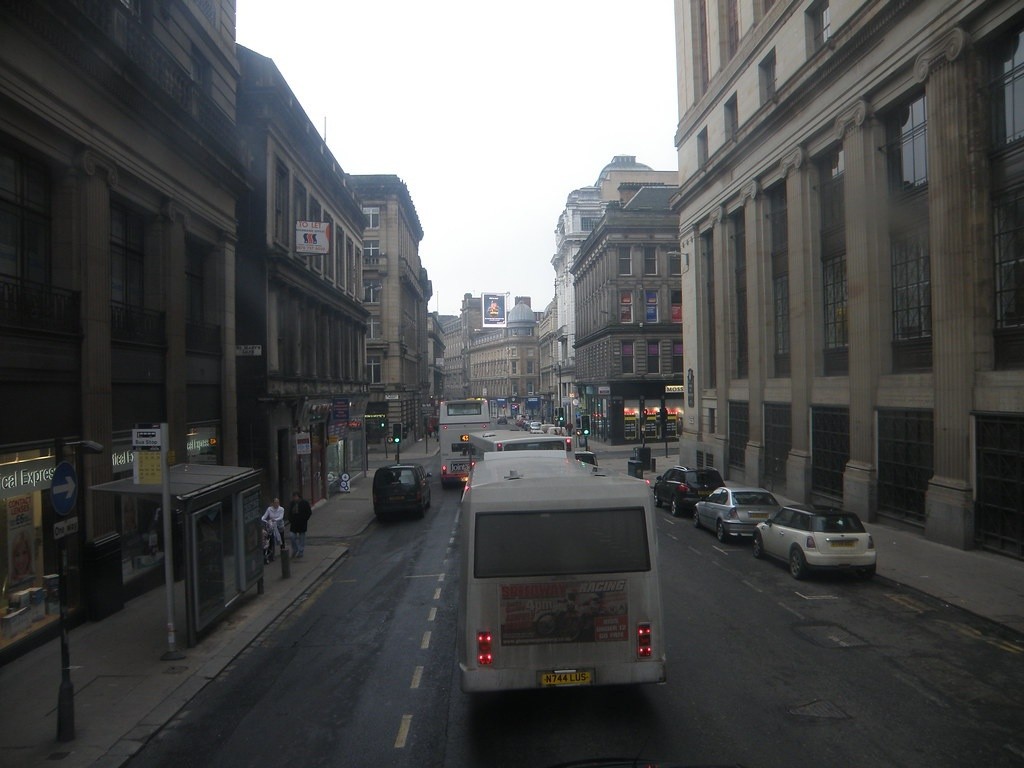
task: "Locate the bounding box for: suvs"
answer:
[653,465,727,517]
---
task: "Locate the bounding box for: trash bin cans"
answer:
[634,447,651,471]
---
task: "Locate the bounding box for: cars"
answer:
[515,414,568,436]
[753,504,876,581]
[497,416,507,424]
[693,487,783,544]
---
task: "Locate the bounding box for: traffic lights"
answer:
[656,409,663,424]
[642,409,647,420]
[581,415,591,436]
[393,423,402,443]
[380,420,386,432]
[558,407,565,421]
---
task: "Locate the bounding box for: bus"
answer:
[459,449,666,695]
[438,400,490,489]
[462,431,577,470]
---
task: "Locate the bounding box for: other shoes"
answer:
[296,550,304,558]
[291,549,298,558]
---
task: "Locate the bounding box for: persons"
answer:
[427,423,434,438]
[287,491,312,560]
[261,497,286,565]
[565,421,573,437]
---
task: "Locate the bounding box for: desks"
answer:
[2,572,60,637]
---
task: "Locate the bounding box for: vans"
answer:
[573,451,598,467]
[372,464,432,524]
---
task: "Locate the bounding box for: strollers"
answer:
[262,521,277,565]
